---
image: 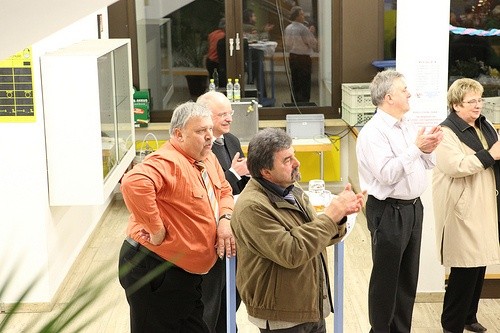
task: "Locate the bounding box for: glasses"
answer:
[216,110,234,118]
[463,98,484,106]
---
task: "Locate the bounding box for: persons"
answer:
[432,78,500,333]
[242,9,273,34]
[118,103,236,333]
[449,0,500,73]
[230,129,368,333]
[284,7,317,102]
[197,93,250,333]
[356,70,443,333]
[206,17,226,88]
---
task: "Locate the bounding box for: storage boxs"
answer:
[341,82,376,126]
[134,90,150,125]
[481,96,500,124]
[286,113,325,138]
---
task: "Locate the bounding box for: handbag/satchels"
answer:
[131,133,159,167]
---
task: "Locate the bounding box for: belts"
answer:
[125,236,183,271]
[388,198,420,204]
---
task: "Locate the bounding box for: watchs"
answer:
[219,214,232,220]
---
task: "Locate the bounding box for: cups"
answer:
[309,180,332,212]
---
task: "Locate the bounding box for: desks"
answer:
[242,136,331,179]
[225,190,355,333]
[247,41,277,107]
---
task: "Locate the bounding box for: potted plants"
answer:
[180,34,209,97]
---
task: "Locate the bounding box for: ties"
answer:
[216,139,223,146]
[284,191,299,208]
[195,161,219,226]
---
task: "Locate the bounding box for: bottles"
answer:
[226,79,234,101]
[213,68,219,88]
[209,79,215,91]
[234,79,241,100]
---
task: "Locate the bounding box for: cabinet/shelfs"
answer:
[137,17,175,111]
[41,38,137,211]
